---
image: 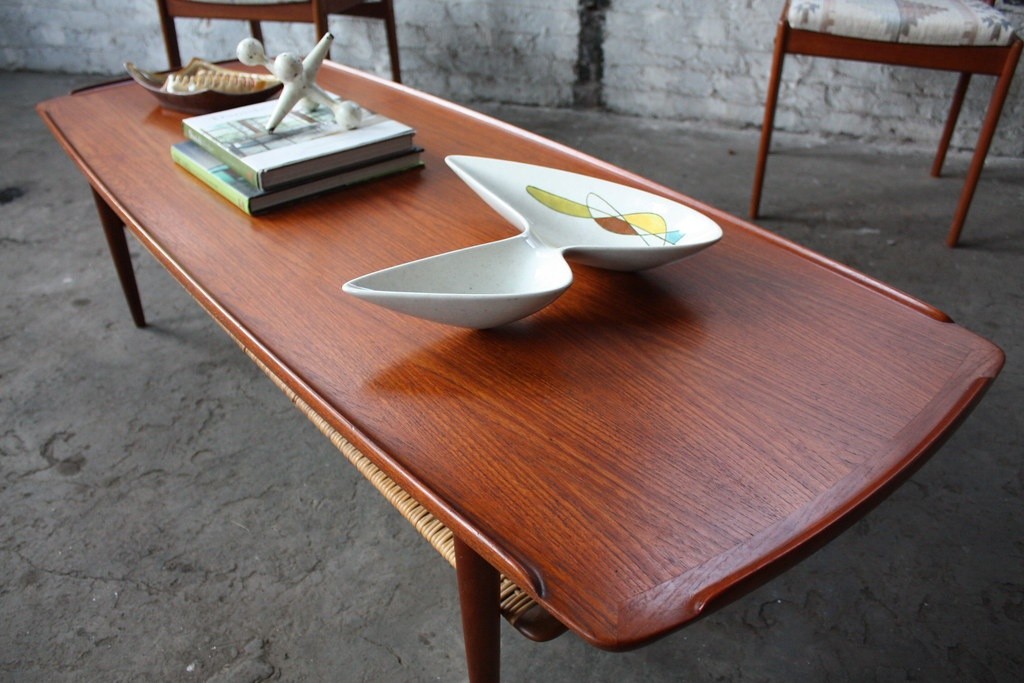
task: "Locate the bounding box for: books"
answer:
[171,89,426,217]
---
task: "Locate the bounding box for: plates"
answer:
[123,57,283,115]
[343,155,724,329]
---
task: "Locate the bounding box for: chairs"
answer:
[748,0,1024,249]
[157,0,403,87]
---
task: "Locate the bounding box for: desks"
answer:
[36,56,1004,683]
